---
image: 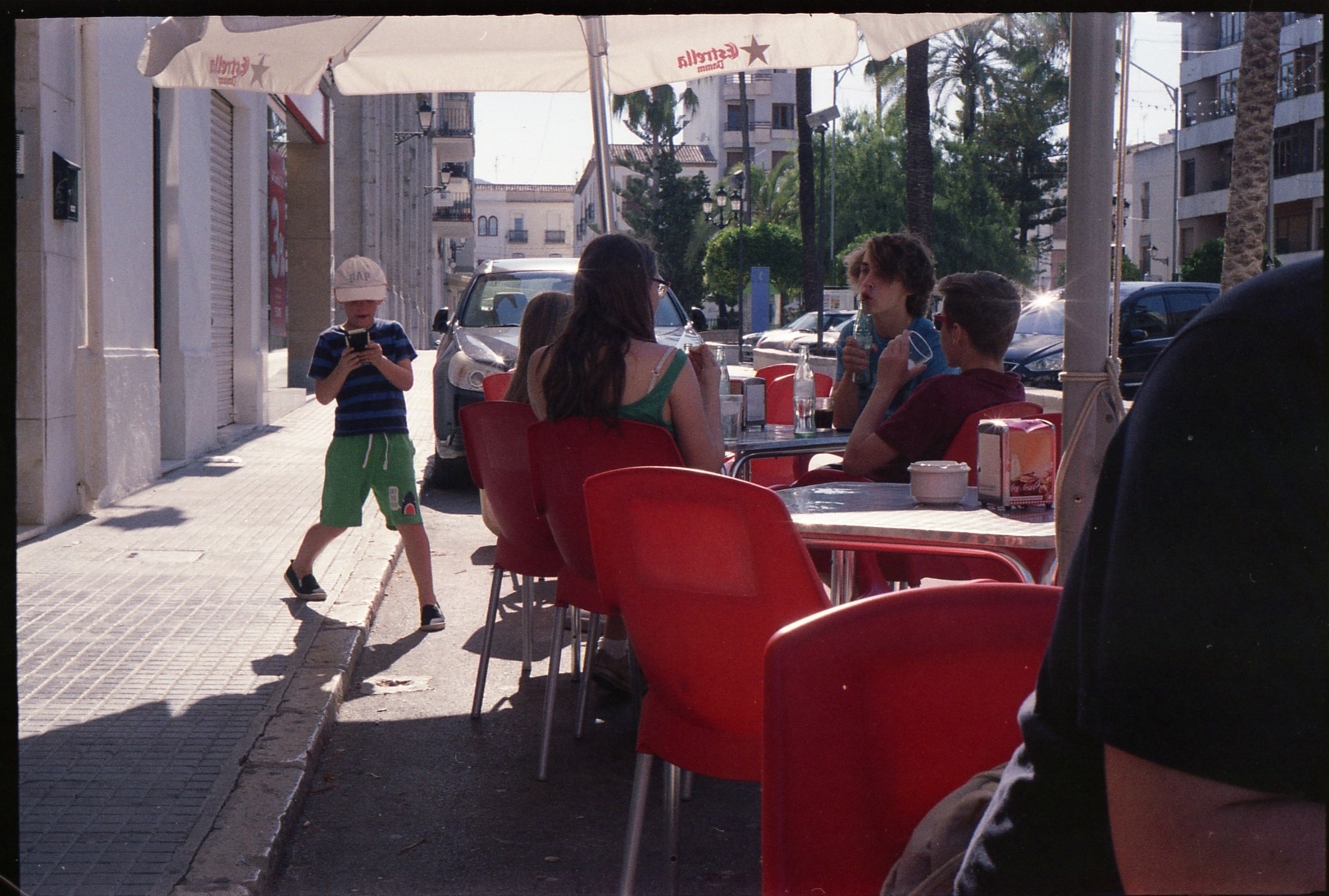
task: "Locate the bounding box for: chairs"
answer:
[457,364,1063,896]
[486,291,529,325]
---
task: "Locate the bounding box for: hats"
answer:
[333,255,386,303]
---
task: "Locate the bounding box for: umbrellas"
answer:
[136,13,995,229]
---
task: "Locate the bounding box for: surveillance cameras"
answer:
[805,105,841,128]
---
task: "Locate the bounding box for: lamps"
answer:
[394,99,435,147]
[423,163,452,195]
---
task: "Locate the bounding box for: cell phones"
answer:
[345,328,370,353]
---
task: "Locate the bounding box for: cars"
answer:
[740,309,861,362]
[432,259,708,490]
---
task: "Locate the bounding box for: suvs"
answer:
[1000,281,1221,402]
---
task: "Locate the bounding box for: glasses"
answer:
[932,312,955,333]
[648,275,677,300]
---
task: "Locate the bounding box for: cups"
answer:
[719,394,744,441]
[907,460,972,504]
[815,397,834,432]
[907,330,933,370]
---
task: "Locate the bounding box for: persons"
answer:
[949,253,1323,896]
[499,233,1027,696]
[284,255,448,631]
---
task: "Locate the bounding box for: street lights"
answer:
[702,185,741,329]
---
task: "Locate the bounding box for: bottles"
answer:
[715,346,730,394]
[684,343,693,354]
[793,345,816,434]
[852,300,874,385]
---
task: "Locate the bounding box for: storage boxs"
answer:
[977,417,1057,512]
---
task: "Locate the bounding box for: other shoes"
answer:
[561,603,609,633]
[284,560,326,600]
[419,602,445,630]
[589,648,647,693]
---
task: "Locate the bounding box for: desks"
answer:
[723,420,851,482]
[771,481,1057,605]
